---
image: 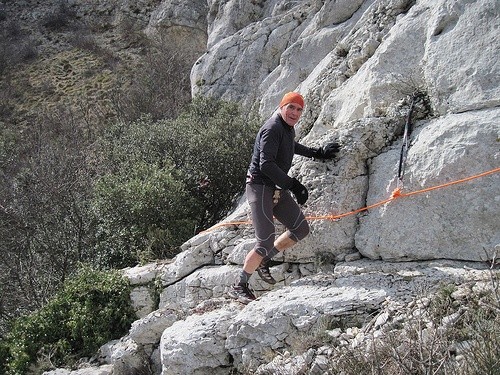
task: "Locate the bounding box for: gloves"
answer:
[286,177,308,205]
[310,142,341,159]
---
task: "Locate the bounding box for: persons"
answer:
[233,92,343,301]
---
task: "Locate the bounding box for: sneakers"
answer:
[228,280,256,305]
[255,257,277,285]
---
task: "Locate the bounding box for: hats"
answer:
[279,91,304,109]
